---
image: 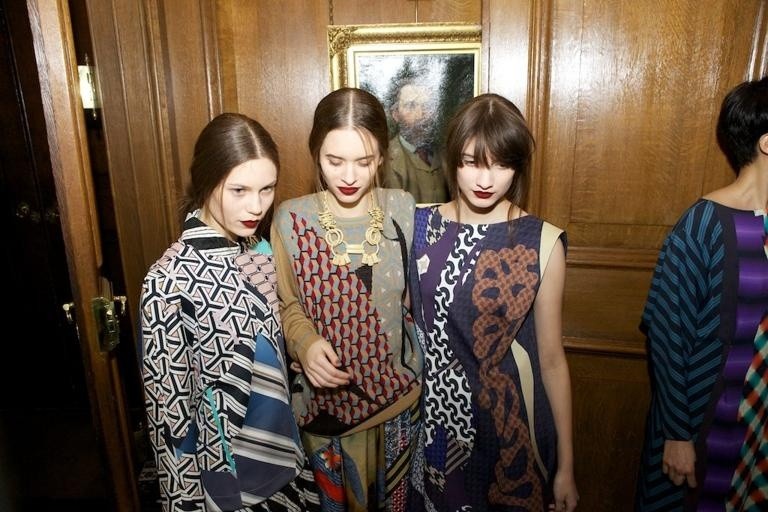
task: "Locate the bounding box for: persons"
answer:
[267,86,424,511]
[633,78,767,512]
[386,78,452,204]
[406,93,580,511]
[136,111,322,512]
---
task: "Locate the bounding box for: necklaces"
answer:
[316,183,385,266]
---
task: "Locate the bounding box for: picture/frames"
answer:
[325,20,483,208]
